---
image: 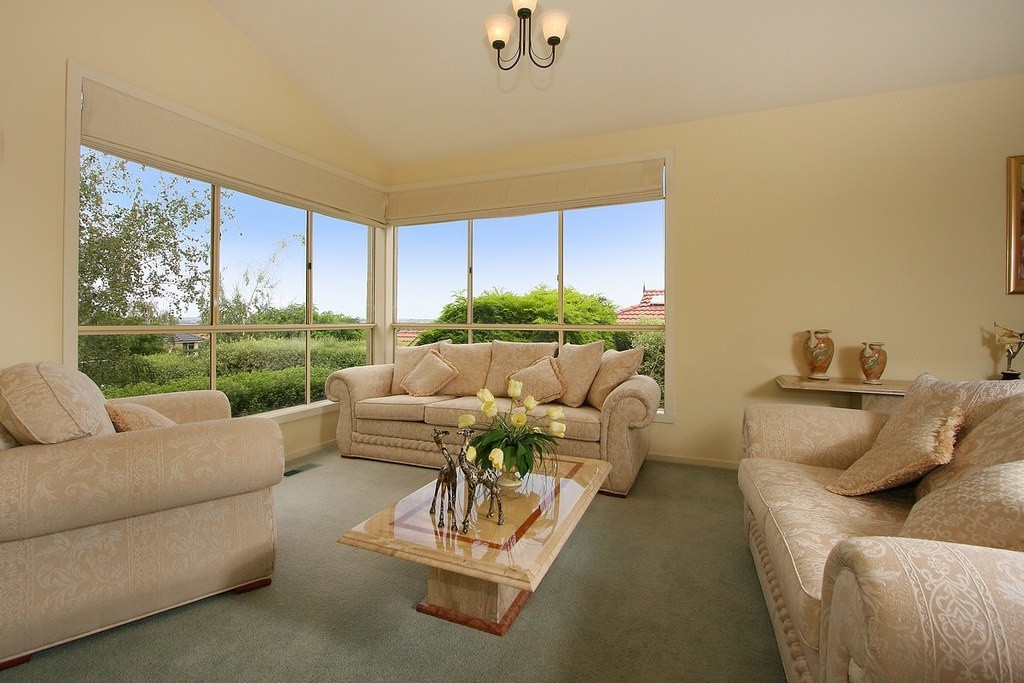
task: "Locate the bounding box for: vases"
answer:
[497,461,520,487]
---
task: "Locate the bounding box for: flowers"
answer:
[458,383,564,494]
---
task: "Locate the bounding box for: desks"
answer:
[776,375,913,413]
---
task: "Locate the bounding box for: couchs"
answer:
[323,363,662,498]
[0,359,285,669]
[736,401,1024,683]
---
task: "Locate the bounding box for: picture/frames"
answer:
[1006,155,1024,295]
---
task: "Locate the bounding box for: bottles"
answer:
[859,340,888,385]
[803,328,835,380]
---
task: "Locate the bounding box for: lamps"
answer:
[484,0,571,71]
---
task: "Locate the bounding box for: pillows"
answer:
[434,342,493,397]
[503,356,566,406]
[392,339,453,393]
[399,349,459,397]
[823,370,1024,553]
[555,339,605,408]
[586,347,644,412]
[486,339,559,396]
[104,402,176,432]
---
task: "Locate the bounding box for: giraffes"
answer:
[458,423,506,535]
[428,427,458,532]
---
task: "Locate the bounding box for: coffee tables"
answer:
[338,451,614,637]
